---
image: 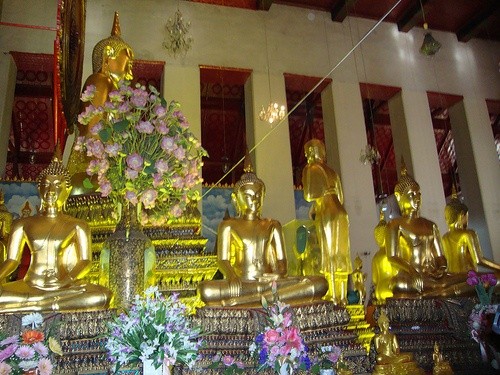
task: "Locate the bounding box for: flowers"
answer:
[75,80,210,227]
[0,311,65,375]
[207,349,247,375]
[248,280,347,375]
[93,285,212,375]
[466,270,500,363]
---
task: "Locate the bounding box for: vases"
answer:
[99,194,157,311]
[143,359,168,375]
[276,354,294,375]
[320,368,336,375]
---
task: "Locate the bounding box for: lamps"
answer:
[221,0,231,173]
[354,0,389,212]
[420,0,442,55]
[257,0,286,130]
[161,0,192,60]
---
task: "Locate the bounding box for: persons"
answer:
[371,210,398,300]
[440,197,500,277]
[432,339,450,371]
[351,256,368,304]
[68,10,134,193]
[0,139,112,314]
[383,154,468,292]
[372,313,413,365]
[302,138,354,303]
[197,149,329,307]
